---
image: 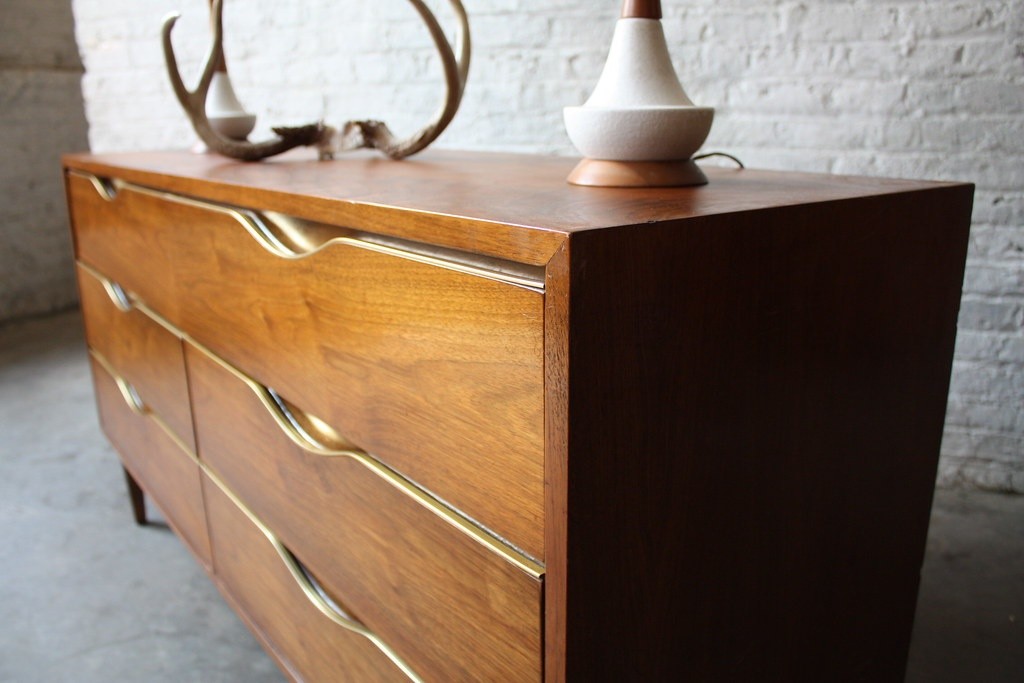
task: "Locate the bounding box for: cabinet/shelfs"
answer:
[62,143,975,683]
